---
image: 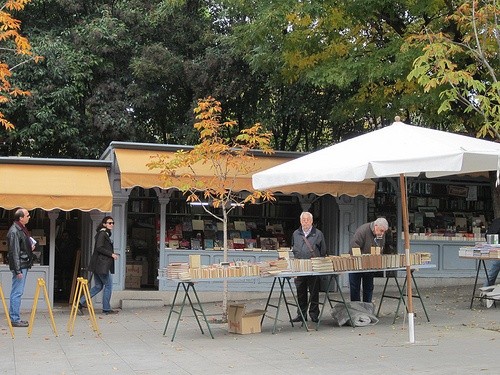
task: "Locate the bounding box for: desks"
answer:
[459,255,500,310]
[157,276,292,342]
[317,263,436,333]
[261,273,353,333]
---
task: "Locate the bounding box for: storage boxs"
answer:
[126,227,153,290]
[228,304,267,334]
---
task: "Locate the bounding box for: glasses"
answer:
[105,221,115,226]
[22,214,30,217]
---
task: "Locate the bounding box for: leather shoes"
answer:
[310,316,319,323]
[290,314,307,323]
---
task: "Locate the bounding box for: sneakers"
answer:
[10,320,29,328]
[72,301,84,316]
[101,308,120,315]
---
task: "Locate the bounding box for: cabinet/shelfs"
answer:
[128,195,261,229]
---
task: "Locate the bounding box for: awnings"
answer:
[0,164,113,213]
[111,148,376,200]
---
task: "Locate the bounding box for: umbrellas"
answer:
[251,120,500,343]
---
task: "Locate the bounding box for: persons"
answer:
[75,216,118,315]
[6,208,34,327]
[482,216,500,289]
[348,217,389,311]
[290,211,326,324]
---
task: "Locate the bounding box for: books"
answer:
[127,181,500,283]
[0,225,46,264]
